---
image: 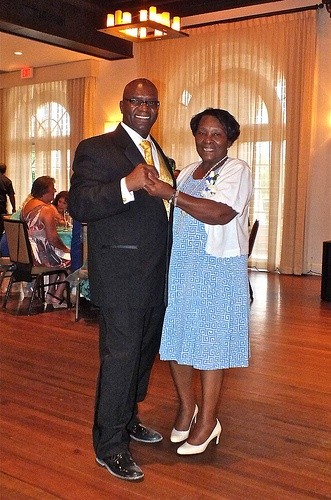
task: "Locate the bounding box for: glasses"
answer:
[123,98,160,109]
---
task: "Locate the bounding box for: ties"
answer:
[141,139,154,166]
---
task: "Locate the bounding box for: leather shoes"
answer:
[129,424,163,442]
[96,448,144,481]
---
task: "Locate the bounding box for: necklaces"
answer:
[179,155,228,213]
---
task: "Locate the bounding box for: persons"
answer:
[0,160,16,244]
[52,190,72,225]
[0,192,34,295]
[66,77,176,480]
[20,175,75,308]
[142,107,253,455]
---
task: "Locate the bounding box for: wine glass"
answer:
[63,209,73,231]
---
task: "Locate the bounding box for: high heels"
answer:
[170,405,200,443]
[177,419,222,455]
[52,295,69,308]
[45,292,53,304]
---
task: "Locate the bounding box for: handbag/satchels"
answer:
[13,263,32,282]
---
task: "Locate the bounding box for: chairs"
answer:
[0,211,260,323]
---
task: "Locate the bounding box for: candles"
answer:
[106,6,181,39]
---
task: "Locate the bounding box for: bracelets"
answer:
[168,190,179,206]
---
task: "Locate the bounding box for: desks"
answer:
[49,223,92,302]
